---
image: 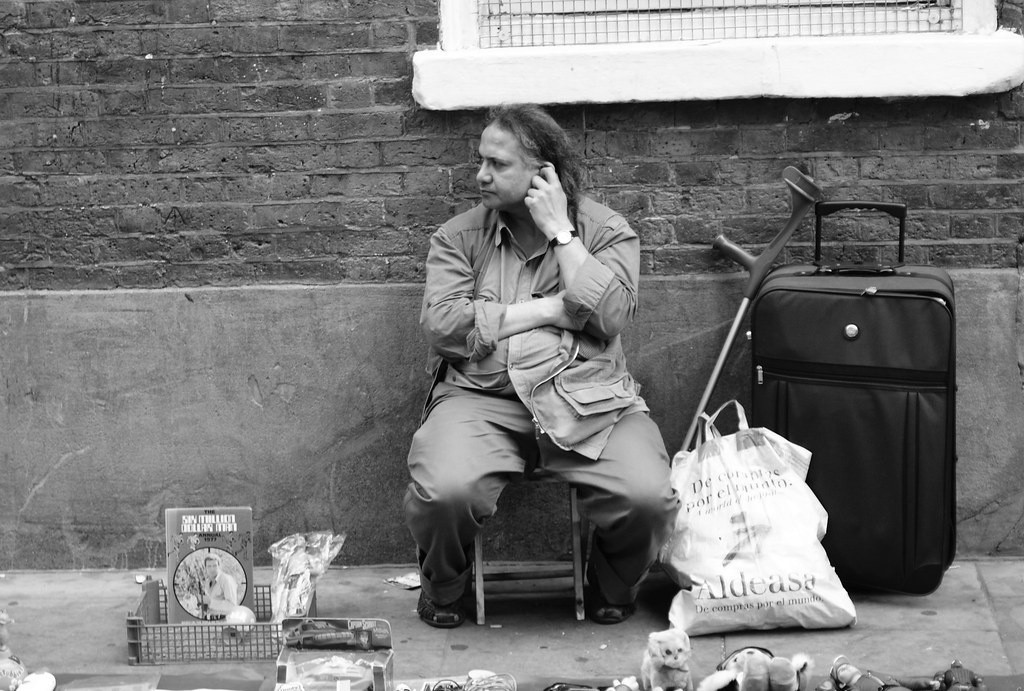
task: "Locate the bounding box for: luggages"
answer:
[751,199,957,595]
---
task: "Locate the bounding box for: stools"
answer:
[470,476,587,627]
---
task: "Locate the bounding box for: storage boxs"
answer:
[124,574,317,666]
[274,617,394,691]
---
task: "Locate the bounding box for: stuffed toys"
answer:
[640,627,693,691]
[696,647,812,691]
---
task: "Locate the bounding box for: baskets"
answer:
[126,578,318,665]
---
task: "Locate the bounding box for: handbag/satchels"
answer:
[659,400,857,638]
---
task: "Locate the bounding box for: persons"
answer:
[934,659,987,691]
[403,106,682,628]
[815,655,941,691]
[203,554,238,619]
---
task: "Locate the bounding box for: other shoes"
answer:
[417,586,467,627]
[590,598,639,624]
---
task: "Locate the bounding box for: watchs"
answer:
[550,230,579,248]
